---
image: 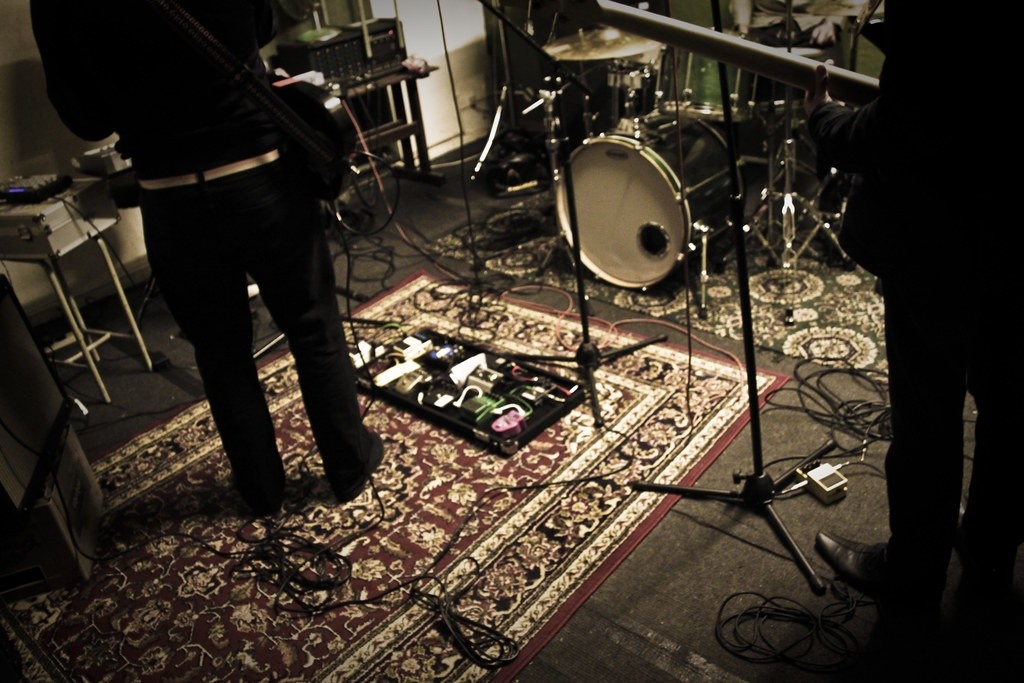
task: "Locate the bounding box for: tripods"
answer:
[476,0,857,595]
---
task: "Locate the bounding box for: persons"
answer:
[798,0,1023,616]
[24,0,383,512]
[727,0,873,272]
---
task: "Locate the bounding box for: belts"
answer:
[140,150,280,190]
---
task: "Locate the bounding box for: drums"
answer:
[663,101,754,166]
[738,46,832,110]
[555,112,746,291]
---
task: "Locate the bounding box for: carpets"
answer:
[0,267,793,683]
[427,185,891,378]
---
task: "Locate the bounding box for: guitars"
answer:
[561,0,879,105]
[264,70,355,201]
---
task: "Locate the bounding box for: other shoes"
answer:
[335,430,384,502]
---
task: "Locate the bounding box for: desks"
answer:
[0,186,153,404]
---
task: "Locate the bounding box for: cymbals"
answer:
[541,27,663,61]
[754,0,866,17]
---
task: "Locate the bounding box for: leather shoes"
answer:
[952,501,1014,594]
[815,532,944,610]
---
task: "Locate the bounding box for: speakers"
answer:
[0,274,69,515]
